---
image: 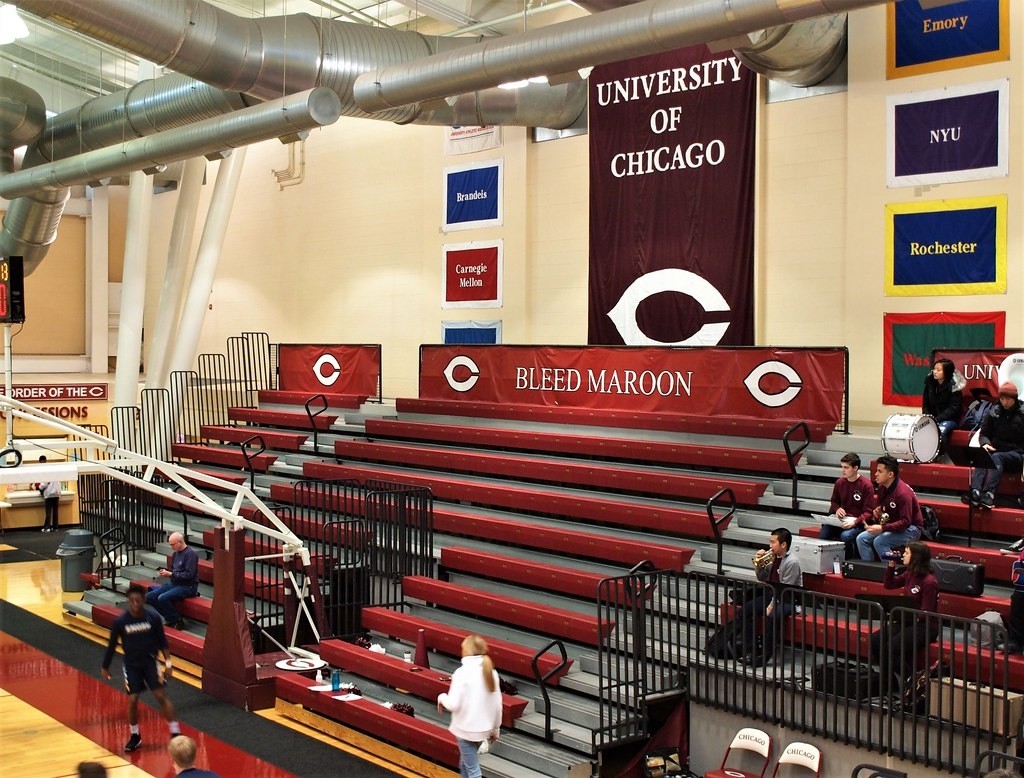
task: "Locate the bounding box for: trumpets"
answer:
[751,549,777,569]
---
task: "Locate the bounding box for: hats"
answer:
[999,382,1019,398]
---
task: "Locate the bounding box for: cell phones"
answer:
[440,677,451,680]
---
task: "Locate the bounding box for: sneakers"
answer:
[979,494,995,508]
[960,491,979,506]
[125,732,142,752]
[1000,538,1024,555]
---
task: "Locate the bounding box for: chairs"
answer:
[771,741,822,778]
[705,727,771,778]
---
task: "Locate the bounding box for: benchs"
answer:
[59,389,1024,778]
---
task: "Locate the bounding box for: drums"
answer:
[881,413,942,464]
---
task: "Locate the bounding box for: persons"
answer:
[437,636,503,778]
[998,537,1024,652]
[144,532,199,631]
[29,455,61,532]
[100,584,182,750]
[78,762,106,778]
[871,541,940,692]
[818,452,874,561]
[738,527,803,667]
[960,380,1024,510]
[921,358,967,462]
[168,735,222,778]
[857,453,924,562]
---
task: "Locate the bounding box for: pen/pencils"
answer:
[346,696,349,698]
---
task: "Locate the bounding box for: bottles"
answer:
[316,669,322,683]
[404,649,411,663]
[881,552,903,560]
[332,671,340,692]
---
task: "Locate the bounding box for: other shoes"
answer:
[738,654,753,665]
[164,617,185,632]
[50,527,60,532]
[41,528,51,532]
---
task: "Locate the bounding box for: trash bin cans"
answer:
[56,529,98,592]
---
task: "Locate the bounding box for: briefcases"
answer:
[841,560,905,584]
[929,555,985,595]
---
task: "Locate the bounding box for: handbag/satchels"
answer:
[644,747,682,777]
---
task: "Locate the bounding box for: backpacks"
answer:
[919,505,941,541]
[901,660,953,716]
[970,611,1006,650]
[962,398,992,432]
